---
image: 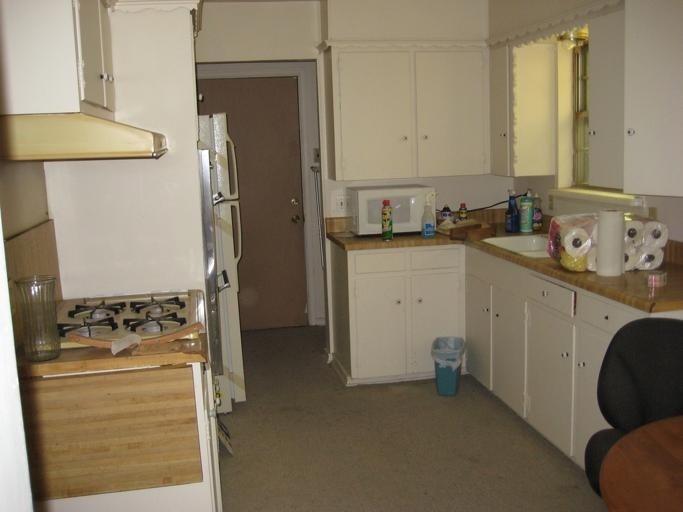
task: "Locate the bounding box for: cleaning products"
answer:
[504,189,518,232]
[380,199,394,242]
[421,193,437,239]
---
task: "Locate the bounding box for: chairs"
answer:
[582,316,683,497]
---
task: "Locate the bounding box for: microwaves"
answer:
[346,184,437,236]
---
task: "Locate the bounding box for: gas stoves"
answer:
[123,311,187,332]
[68,299,127,321]
[130,296,186,314]
[57,317,119,337]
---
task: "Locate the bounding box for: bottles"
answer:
[442,204,450,218]
[382,200,393,242]
[520,198,533,232]
[505,195,518,233]
[422,206,435,238]
[459,203,467,221]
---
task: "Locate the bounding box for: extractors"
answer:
[0,113,169,161]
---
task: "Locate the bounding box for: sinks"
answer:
[480,233,552,258]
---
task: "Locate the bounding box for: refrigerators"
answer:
[199,112,246,417]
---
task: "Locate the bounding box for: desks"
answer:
[595,413,682,511]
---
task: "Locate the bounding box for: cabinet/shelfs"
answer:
[3,0,117,123]
[492,38,557,178]
[585,1,683,199]
[328,234,467,388]
[470,244,683,472]
[316,38,492,182]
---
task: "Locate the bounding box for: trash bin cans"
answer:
[431,337,466,396]
